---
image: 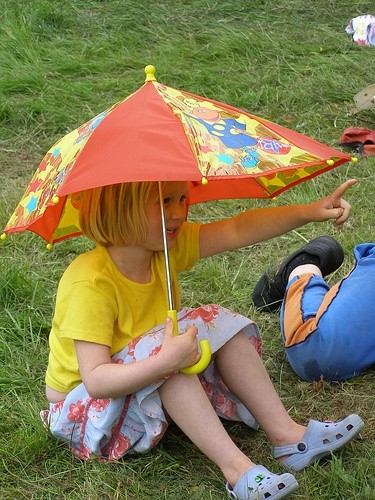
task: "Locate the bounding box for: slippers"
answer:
[272,414,365,471]
[226,464,300,500]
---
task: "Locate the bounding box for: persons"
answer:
[252,237,375,382]
[39,178,365,500]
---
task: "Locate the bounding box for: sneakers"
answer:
[252,236,344,313]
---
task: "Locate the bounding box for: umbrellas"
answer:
[0,65,359,375]
